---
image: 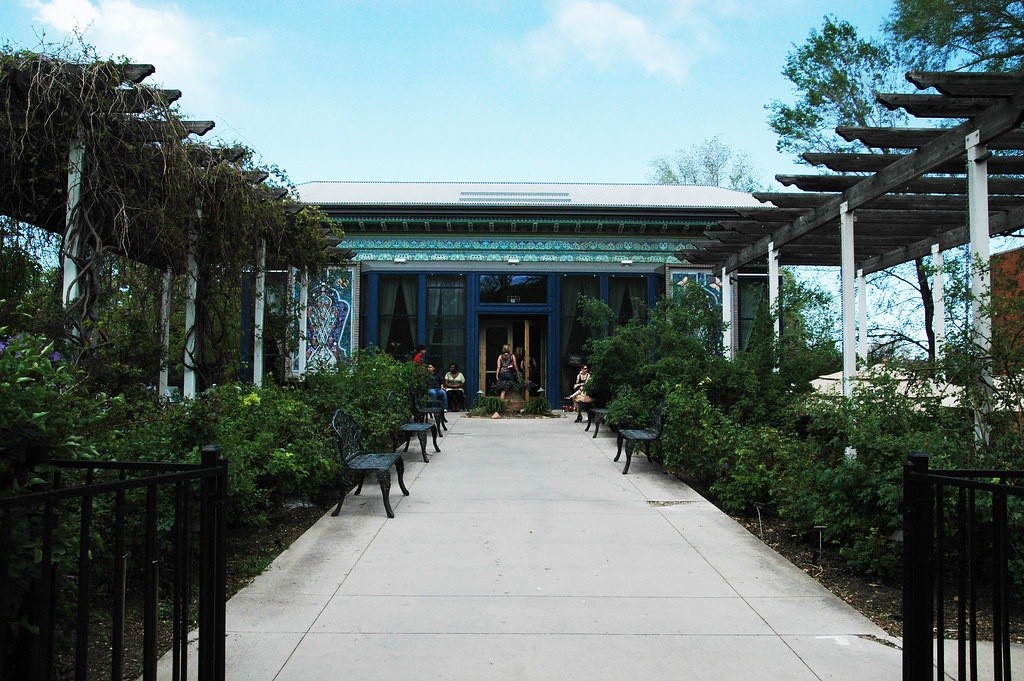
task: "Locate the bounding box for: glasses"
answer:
[583,368,588,370]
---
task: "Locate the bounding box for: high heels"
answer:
[575,414,583,423]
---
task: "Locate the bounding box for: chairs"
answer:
[585,382,622,438]
[330,409,409,519]
[387,390,442,464]
[613,401,666,474]
[410,388,448,437]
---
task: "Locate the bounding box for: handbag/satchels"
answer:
[507,354,514,370]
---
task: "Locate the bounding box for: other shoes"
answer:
[444,409,448,413]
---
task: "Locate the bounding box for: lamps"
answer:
[507,259,519,265]
[620,260,632,267]
[393,258,405,264]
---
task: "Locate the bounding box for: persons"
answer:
[413,345,426,366]
[426,364,448,413]
[563,364,590,423]
[520,355,536,399]
[445,363,465,412]
[495,344,520,401]
